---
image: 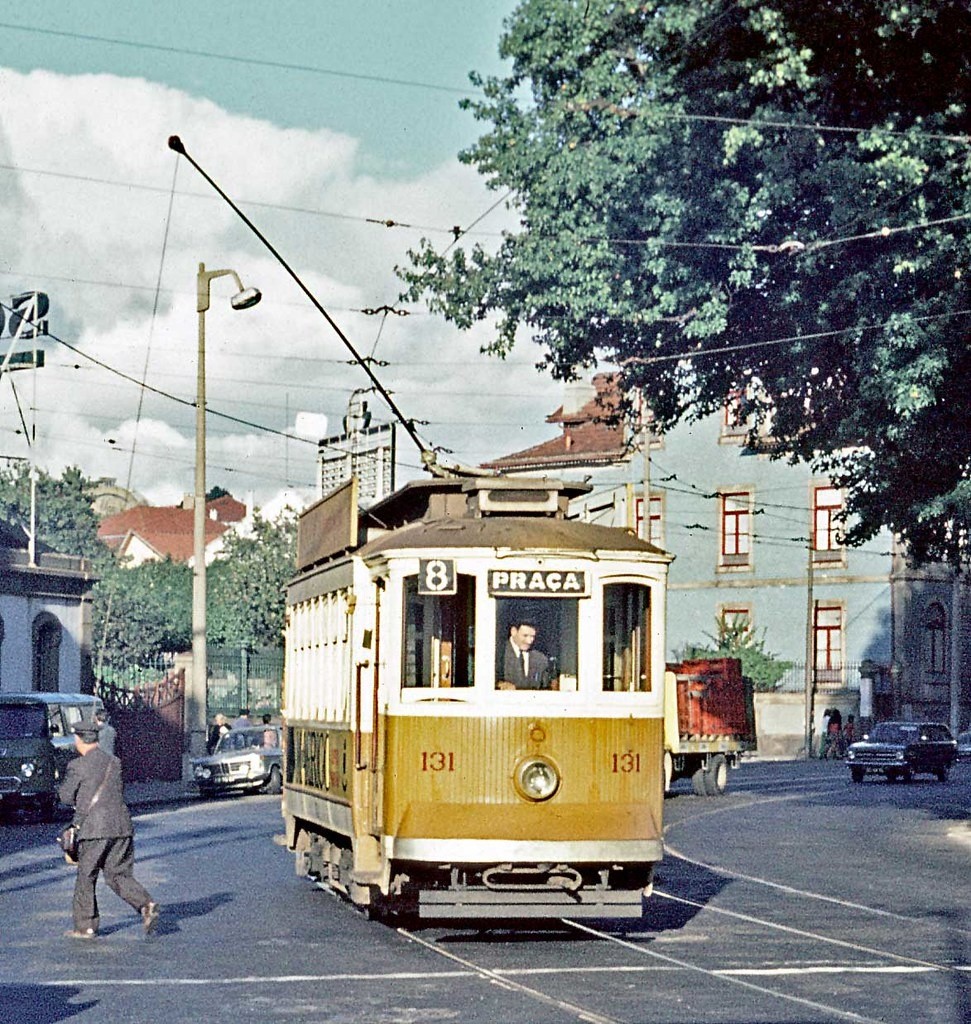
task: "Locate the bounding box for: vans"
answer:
[0,692,109,819]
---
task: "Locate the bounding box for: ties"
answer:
[518,651,525,678]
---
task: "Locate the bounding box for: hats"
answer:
[70,722,100,736]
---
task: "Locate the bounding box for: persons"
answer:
[58,722,160,939]
[495,615,550,690]
[95,708,118,756]
[819,708,856,761]
[206,709,272,756]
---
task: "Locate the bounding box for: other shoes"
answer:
[140,902,160,934]
[65,929,96,939]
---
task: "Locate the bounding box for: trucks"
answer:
[663,655,758,798]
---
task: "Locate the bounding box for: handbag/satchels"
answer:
[58,825,77,862]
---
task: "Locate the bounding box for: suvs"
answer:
[844,720,960,785]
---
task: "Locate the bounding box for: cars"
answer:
[956,734,970,757]
[189,725,283,795]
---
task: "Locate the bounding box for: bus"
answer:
[167,134,675,926]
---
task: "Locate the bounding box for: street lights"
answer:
[185,260,261,792]
